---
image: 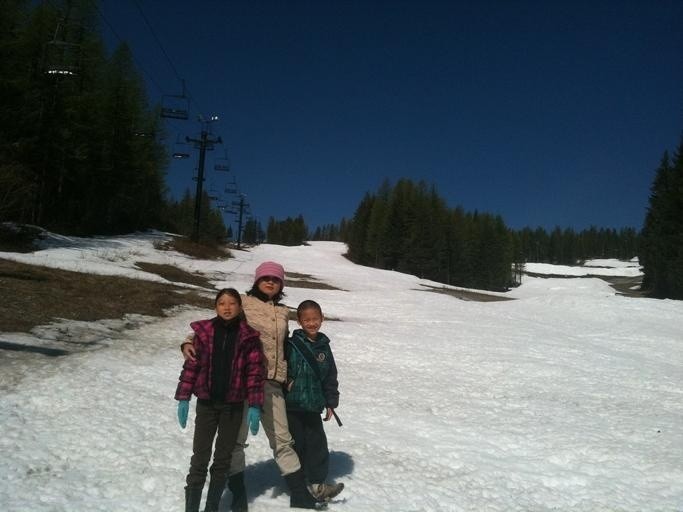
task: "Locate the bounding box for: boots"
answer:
[285,466,328,510]
[228,470,247,512]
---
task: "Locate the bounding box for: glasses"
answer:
[260,276,281,284]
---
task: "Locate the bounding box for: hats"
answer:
[253,261,284,292]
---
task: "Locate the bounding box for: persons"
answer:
[180,262,327,512]
[285,299,344,502]
[174,288,265,512]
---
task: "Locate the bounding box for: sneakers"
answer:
[305,483,344,502]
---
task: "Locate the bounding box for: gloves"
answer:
[178,399,189,429]
[247,405,261,436]
